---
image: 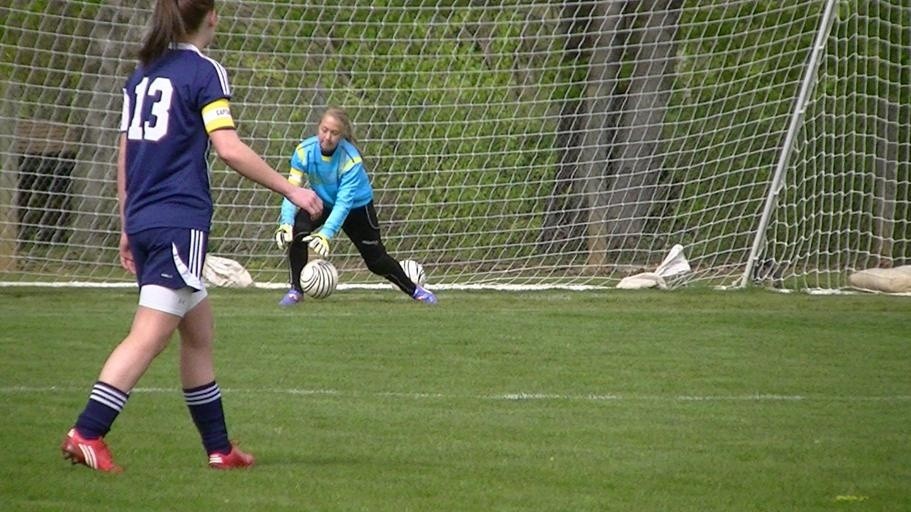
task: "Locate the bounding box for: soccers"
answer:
[393,260,425,293]
[300,259,338,299]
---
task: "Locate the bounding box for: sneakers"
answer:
[278,290,304,305]
[412,285,437,305]
[207,446,255,468]
[61,429,120,473]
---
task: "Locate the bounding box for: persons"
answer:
[274,105,439,308]
[59,0,325,472]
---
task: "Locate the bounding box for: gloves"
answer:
[303,233,330,260]
[274,225,294,249]
